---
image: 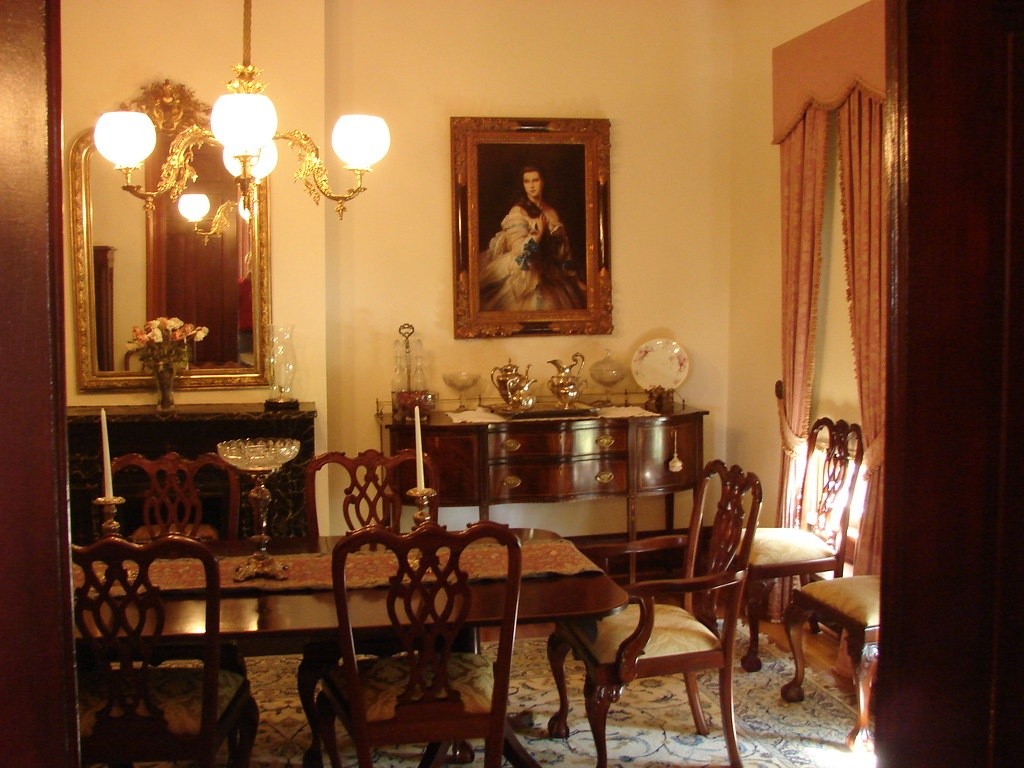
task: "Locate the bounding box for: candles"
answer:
[100,407,113,500]
[414,406,425,488]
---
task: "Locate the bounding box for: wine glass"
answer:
[441,372,477,412]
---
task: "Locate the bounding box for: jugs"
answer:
[547,352,585,407]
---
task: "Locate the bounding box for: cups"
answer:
[547,378,588,410]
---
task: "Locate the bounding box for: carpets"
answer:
[113,620,879,768]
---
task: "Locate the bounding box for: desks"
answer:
[72,526,629,768]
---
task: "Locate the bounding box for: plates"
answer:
[630,339,689,390]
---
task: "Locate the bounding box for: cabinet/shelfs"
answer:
[374,389,708,575]
[68,401,321,554]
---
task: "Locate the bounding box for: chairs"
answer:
[70,452,258,768]
[294,447,524,768]
[729,418,862,672]
[781,573,880,752]
[547,460,763,767]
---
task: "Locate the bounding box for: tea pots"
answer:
[490,359,531,405]
[506,378,537,410]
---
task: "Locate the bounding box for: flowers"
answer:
[127,317,208,408]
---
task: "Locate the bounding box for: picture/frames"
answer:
[449,117,615,341]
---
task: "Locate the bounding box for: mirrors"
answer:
[69,81,273,391]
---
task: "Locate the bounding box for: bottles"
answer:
[265,324,296,404]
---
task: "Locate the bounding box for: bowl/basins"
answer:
[390,389,439,422]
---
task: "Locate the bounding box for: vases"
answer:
[266,323,300,408]
[152,365,176,412]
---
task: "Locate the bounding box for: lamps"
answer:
[93,0,390,220]
[177,193,253,246]
